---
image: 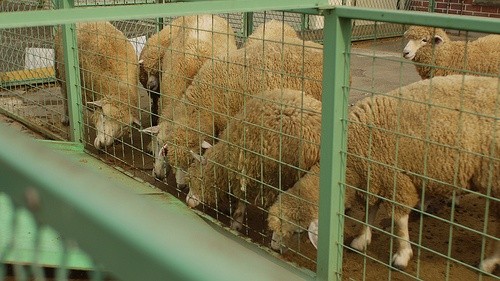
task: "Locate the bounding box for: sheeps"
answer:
[139,14,354,233]
[54,20,142,153]
[402,25,500,79]
[265,74,500,275]
